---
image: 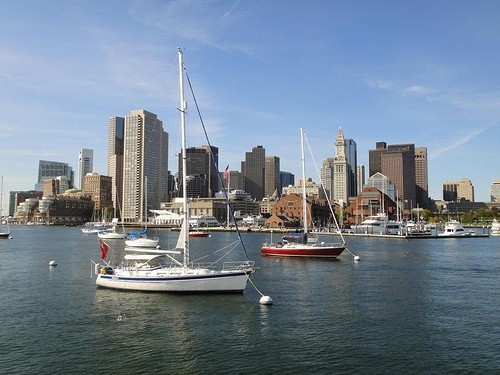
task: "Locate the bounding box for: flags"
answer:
[224,164,229,180]
[98,239,108,260]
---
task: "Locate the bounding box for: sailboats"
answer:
[189,195,209,237]
[90,46,255,292]
[260,126,347,258]
[124,176,160,247]
[97,186,128,238]
[0,174,13,239]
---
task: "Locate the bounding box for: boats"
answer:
[437,219,490,237]
[349,211,408,234]
[80,217,119,234]
